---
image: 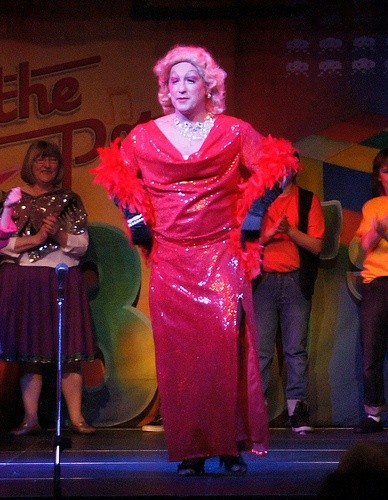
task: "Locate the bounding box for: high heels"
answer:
[219,455,248,476]
[177,457,206,478]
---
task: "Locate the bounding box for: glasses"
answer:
[31,156,58,165]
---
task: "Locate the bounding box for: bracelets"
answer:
[126,213,144,227]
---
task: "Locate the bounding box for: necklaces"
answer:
[175,113,214,144]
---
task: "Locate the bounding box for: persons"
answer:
[0,140,100,437]
[253,151,326,433]
[87,45,304,478]
[354,148,388,434]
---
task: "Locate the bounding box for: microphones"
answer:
[55,263,69,302]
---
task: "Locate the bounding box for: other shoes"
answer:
[10,422,41,436]
[69,420,96,435]
[353,415,383,434]
[289,400,313,433]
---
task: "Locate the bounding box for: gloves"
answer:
[113,195,152,254]
